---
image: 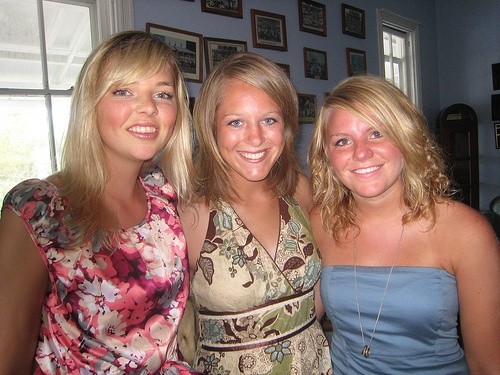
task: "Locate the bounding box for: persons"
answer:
[1,31,195,375]
[307,74,500,375]
[176,51,334,375]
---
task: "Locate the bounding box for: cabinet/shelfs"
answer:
[436,104,479,212]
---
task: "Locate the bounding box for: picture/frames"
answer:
[298,0,327,38]
[303,46,328,80]
[298,93,316,123]
[146,21,204,82]
[249,8,288,50]
[342,2,366,39]
[346,48,368,77]
[201,0,243,21]
[203,34,248,74]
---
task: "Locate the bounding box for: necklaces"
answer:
[352,226,404,359]
